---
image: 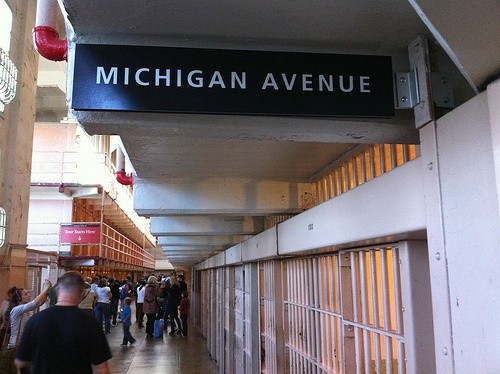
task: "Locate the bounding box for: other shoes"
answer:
[146,334,154,338]
[112,323,117,326]
[106,330,112,334]
[169,332,174,336]
[120,344,128,346]
[177,330,181,334]
[130,340,137,345]
[181,334,186,338]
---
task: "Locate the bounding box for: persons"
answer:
[0,271,190,350]
[14,271,114,374]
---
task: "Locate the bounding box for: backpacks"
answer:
[119,285,126,299]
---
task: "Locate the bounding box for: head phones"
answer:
[16,289,22,302]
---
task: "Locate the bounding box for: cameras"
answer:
[45,279,50,283]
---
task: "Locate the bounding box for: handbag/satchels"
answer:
[0,348,30,374]
[154,320,164,338]
